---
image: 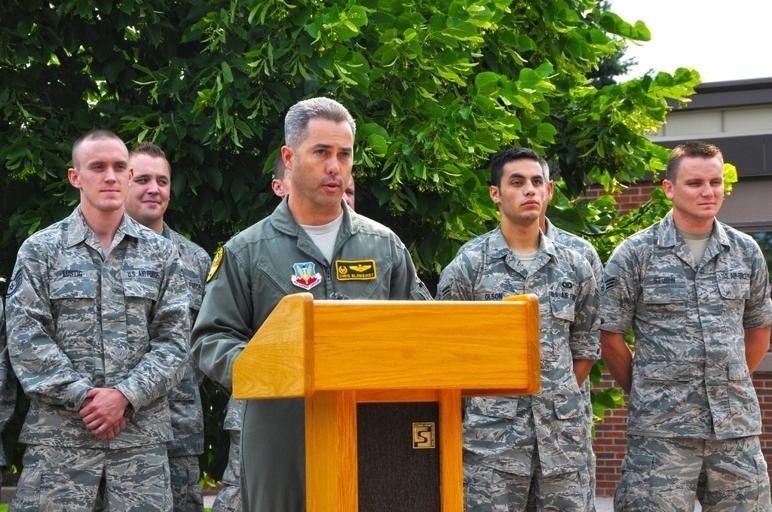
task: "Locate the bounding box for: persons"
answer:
[436,146,604,512]
[598,142,772,512]
[190,97,435,512]
[5,132,212,512]
